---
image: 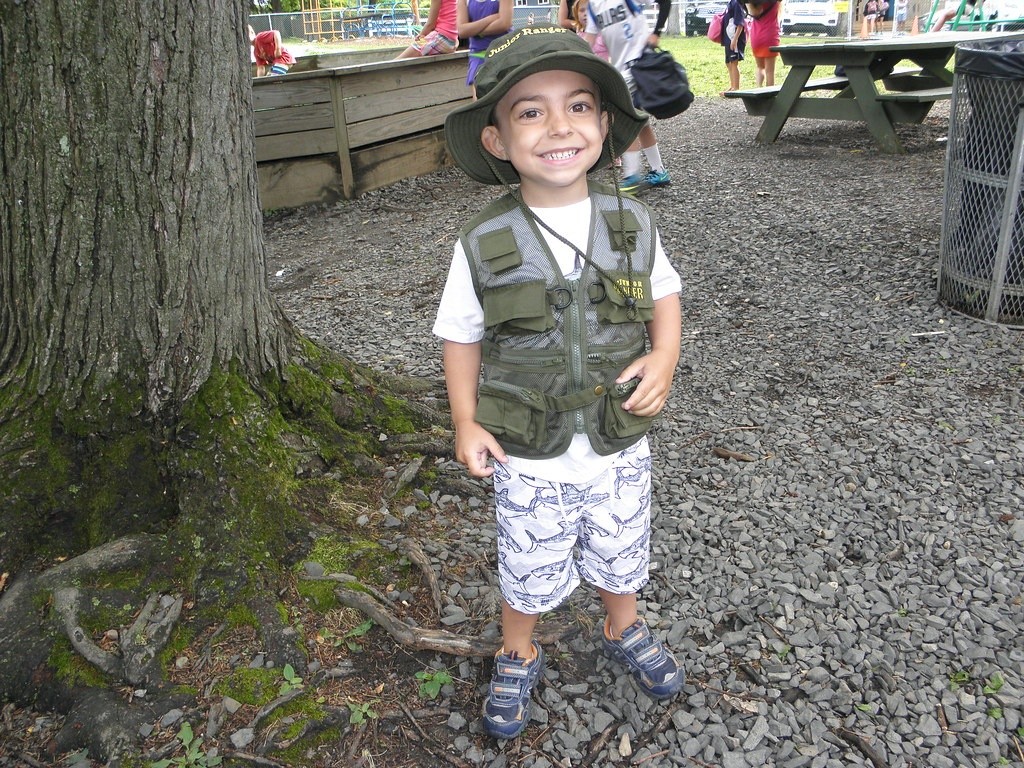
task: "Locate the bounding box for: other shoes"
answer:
[609,157,622,170]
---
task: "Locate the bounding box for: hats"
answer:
[445,21,652,185]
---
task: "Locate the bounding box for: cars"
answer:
[779,0,841,37]
[641,0,730,36]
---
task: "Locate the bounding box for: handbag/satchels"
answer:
[631,45,693,120]
[708,8,728,44]
[879,1,889,11]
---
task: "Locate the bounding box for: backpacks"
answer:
[867,2,877,12]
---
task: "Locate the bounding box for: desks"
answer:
[755,31,1024,155]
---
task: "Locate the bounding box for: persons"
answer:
[249,25,297,78]
[863,0,908,35]
[929,0,986,32]
[392,0,459,60]
[558,0,670,197]
[719,0,782,97]
[456,0,513,102]
[433,22,682,739]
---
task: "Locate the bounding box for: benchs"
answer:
[724,75,849,117]
[874,84,968,124]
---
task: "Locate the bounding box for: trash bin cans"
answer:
[935,34,1024,326]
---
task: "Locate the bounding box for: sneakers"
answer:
[482,639,546,739]
[602,613,686,699]
[644,170,670,186]
[621,179,646,194]
